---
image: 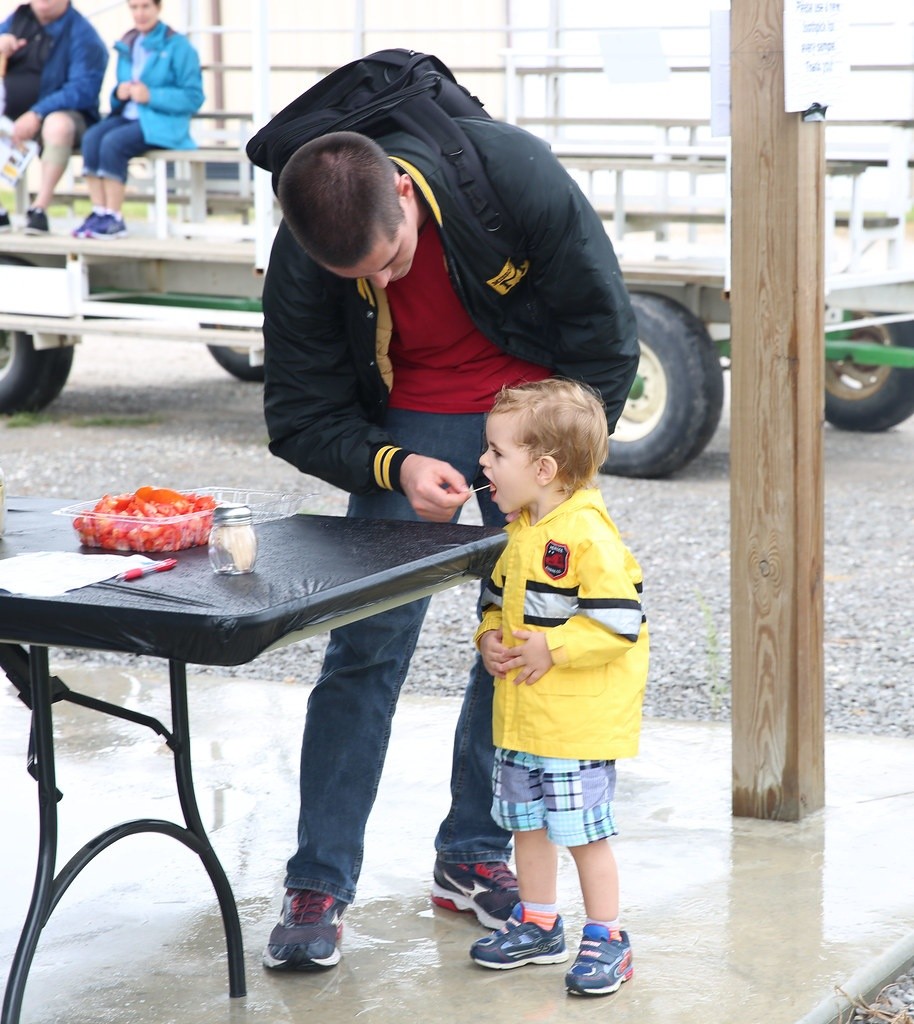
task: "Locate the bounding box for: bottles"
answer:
[207,506,257,574]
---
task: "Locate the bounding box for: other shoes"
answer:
[0,210,13,233]
[72,212,97,238]
[87,215,130,240]
[24,207,51,236]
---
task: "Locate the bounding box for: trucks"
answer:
[0,57,913,480]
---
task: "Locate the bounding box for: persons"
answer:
[0,0,109,236]
[261,114,642,969]
[470,378,650,993]
[72,0,206,240]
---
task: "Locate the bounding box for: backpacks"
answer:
[242,49,534,274]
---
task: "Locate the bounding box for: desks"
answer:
[0,492,510,1024]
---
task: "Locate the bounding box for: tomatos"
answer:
[73,484,216,553]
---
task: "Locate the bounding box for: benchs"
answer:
[17,128,250,240]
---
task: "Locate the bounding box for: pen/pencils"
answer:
[115,556,179,580]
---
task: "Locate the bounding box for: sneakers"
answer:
[431,858,521,930]
[469,902,569,970]
[263,888,344,972]
[565,923,633,995]
